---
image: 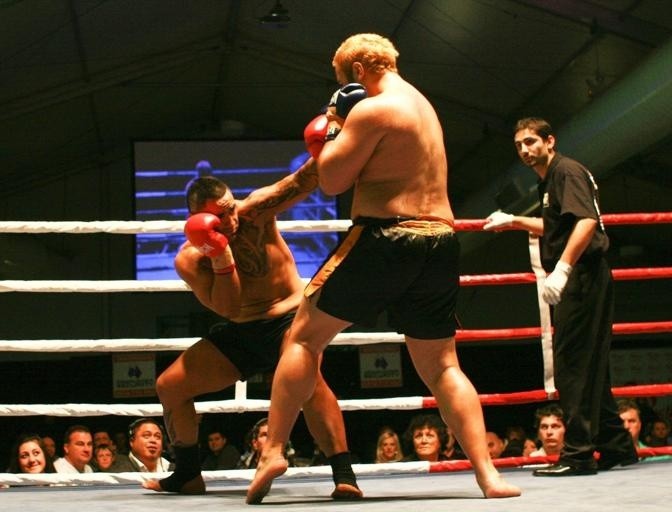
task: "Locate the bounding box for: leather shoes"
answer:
[533,455,597,476]
[596,450,639,471]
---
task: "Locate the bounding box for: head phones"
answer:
[129,418,168,448]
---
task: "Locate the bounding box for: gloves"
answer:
[328,84,365,119]
[305,114,329,159]
[483,211,513,230]
[542,260,573,305]
[185,213,235,274]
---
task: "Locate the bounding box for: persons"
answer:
[376,415,452,465]
[205,417,297,472]
[482,116,641,478]
[144,114,361,499]
[619,399,667,447]
[14,417,169,473]
[244,32,522,504]
[483,402,568,459]
